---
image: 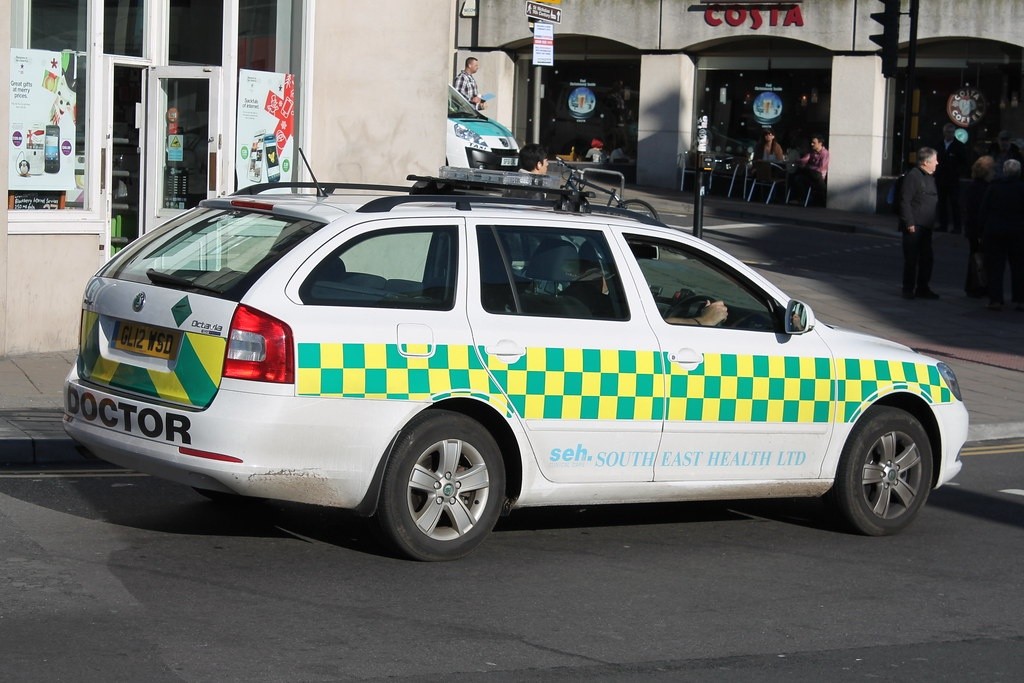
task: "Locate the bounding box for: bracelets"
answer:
[693,318,702,326]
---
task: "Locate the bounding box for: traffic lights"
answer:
[868,1,904,80]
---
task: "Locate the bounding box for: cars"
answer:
[446,83,523,168]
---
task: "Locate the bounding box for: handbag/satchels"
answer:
[974,240,985,286]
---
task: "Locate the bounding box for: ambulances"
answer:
[62,164,970,562]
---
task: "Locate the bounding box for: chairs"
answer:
[318,256,439,308]
[525,243,595,319]
[676,145,827,208]
[480,234,524,305]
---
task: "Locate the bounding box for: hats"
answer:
[1000,131,1011,138]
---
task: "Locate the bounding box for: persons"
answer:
[789,132,829,207]
[965,131,1024,312]
[898,147,940,300]
[454,57,486,110]
[585,139,636,165]
[484,236,728,328]
[502,144,549,200]
[741,128,783,202]
[932,123,966,234]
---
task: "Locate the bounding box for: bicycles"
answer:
[556,155,662,218]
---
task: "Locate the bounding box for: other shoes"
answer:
[916,287,939,300]
[903,288,914,299]
[984,302,1001,311]
[951,228,961,234]
[1009,302,1024,311]
[934,226,947,232]
[967,286,982,297]
